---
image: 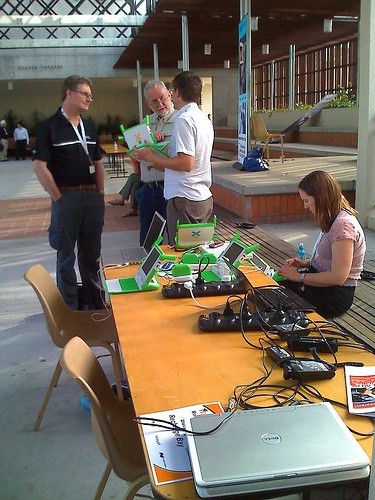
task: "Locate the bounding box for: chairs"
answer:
[251,114,286,164]
[22,264,125,432]
[59,336,148,500]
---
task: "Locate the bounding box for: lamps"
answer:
[204,43,211,55]
[7,81,13,90]
[177,60,183,69]
[224,59,230,69]
[323,18,333,33]
[250,16,259,31]
[262,44,269,55]
[132,79,137,88]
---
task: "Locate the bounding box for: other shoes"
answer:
[123,211,138,217]
[108,199,124,206]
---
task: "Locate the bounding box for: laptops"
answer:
[100,212,166,266]
[123,122,171,153]
[182,401,370,499]
[180,242,248,277]
[103,244,162,294]
[174,223,215,250]
[125,144,171,167]
[221,257,316,313]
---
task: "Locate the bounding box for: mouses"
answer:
[161,261,175,271]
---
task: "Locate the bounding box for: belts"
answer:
[59,184,97,191]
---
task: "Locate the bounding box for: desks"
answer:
[100,143,131,179]
[101,259,375,500]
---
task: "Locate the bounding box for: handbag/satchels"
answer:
[243,146,269,171]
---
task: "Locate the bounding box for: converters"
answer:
[266,346,290,367]
[292,336,338,353]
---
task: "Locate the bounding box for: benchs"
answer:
[206,198,375,356]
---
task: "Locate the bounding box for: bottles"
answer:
[297,243,306,272]
[114,142,117,149]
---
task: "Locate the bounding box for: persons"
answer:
[33,75,105,311]
[276,171,366,318]
[14,122,29,161]
[108,173,143,217]
[0,120,9,162]
[138,80,178,247]
[132,71,214,245]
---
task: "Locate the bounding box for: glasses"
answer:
[169,89,176,94]
[150,97,167,104]
[72,91,93,100]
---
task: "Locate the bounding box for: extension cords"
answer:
[162,282,244,300]
[198,311,308,331]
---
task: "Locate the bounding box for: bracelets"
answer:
[300,273,305,283]
